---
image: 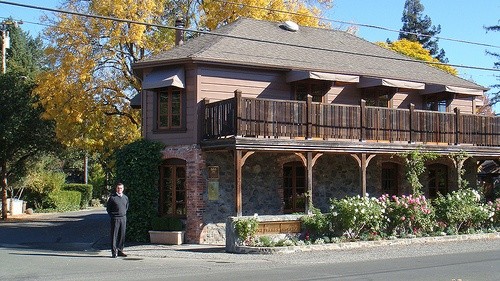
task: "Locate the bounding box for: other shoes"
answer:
[118,252,127,256]
[112,253,117,257]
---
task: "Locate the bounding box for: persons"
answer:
[107,183,130,257]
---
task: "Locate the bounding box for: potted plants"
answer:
[147,215,186,247]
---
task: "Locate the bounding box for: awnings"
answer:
[141,65,186,89]
[286,70,360,85]
[420,85,484,97]
[358,77,425,91]
